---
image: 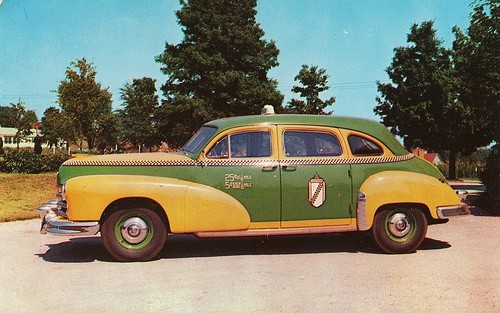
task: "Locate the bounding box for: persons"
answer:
[33,134,42,154]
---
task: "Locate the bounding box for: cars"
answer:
[34,104,471,264]
